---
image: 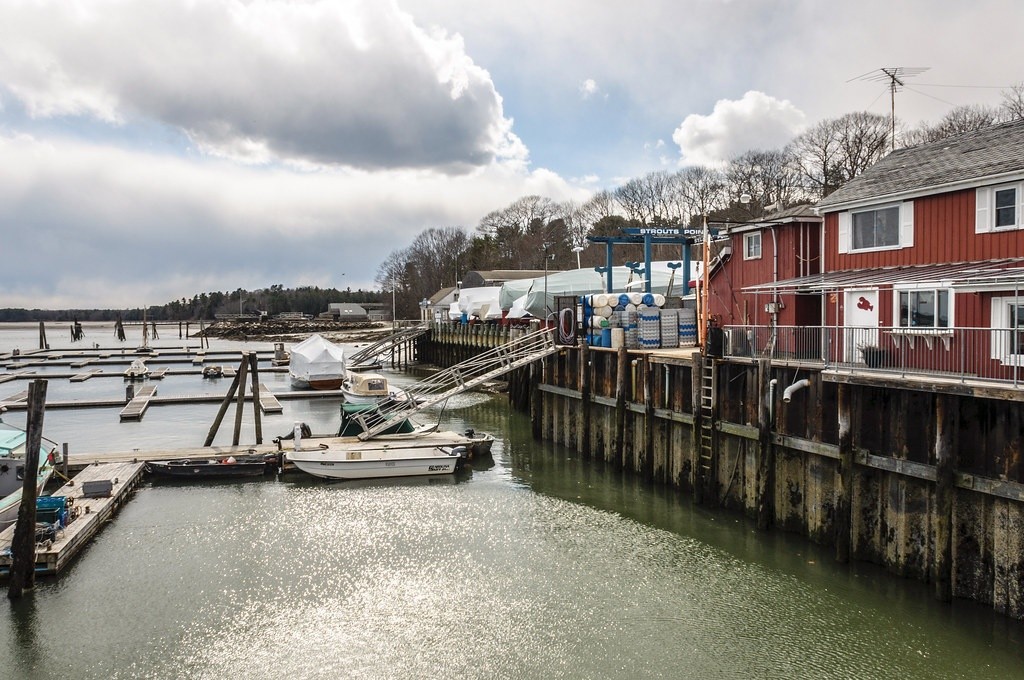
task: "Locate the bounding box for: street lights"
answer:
[571,247,584,269]
[240,300,246,314]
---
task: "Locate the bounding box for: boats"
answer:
[340,374,388,403]
[458,428,496,456]
[123,359,148,379]
[202,366,221,379]
[276,401,439,440]
[0,466,54,533]
[286,446,469,479]
[289,334,347,389]
[148,438,282,477]
